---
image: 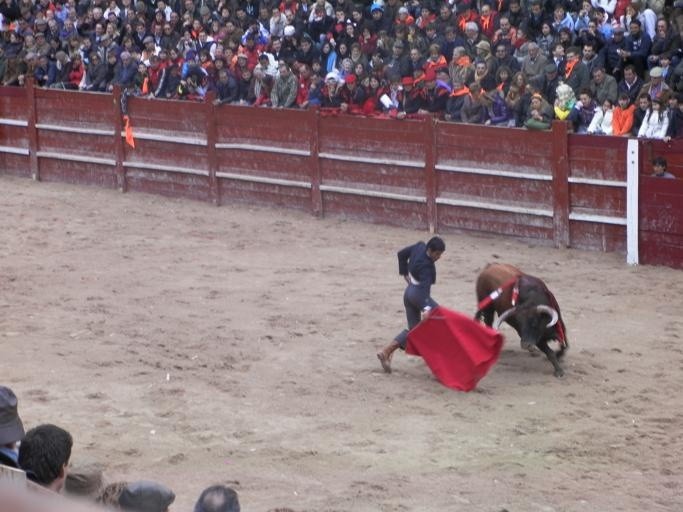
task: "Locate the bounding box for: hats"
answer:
[653,92,664,104]
[142,36,154,44]
[65,464,102,494]
[424,71,435,81]
[0,385,24,445]
[19,6,109,61]
[394,38,403,48]
[284,26,295,37]
[118,479,175,512]
[545,63,557,74]
[402,77,413,85]
[612,28,624,35]
[345,75,356,83]
[474,41,491,52]
[649,66,662,77]
[371,4,384,14]
[200,6,209,16]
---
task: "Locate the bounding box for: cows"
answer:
[474,261,573,379]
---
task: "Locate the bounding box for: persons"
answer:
[1,387,241,512]
[1,0,682,143]
[377,237,444,373]
[650,157,677,178]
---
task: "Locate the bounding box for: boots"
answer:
[376,340,400,373]
